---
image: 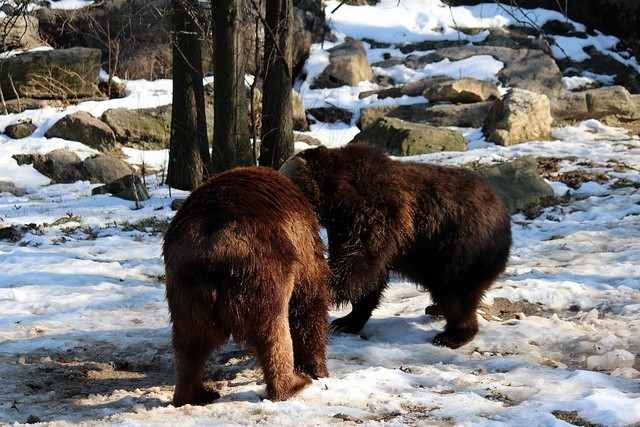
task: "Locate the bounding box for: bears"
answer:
[159,165,333,408]
[279,145,513,350]
